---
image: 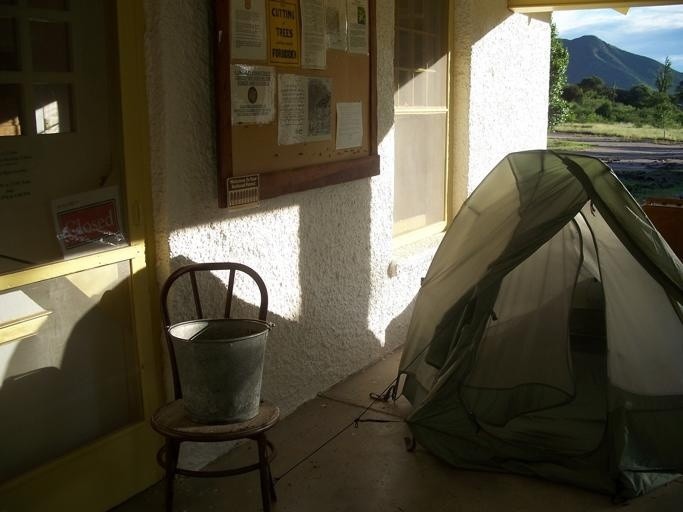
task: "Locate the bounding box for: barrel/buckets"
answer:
[167,319,275,425]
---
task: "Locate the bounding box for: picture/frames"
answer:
[207,0,381,208]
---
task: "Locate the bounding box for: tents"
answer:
[397,150,683,504]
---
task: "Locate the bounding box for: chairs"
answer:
[150,262,279,512]
